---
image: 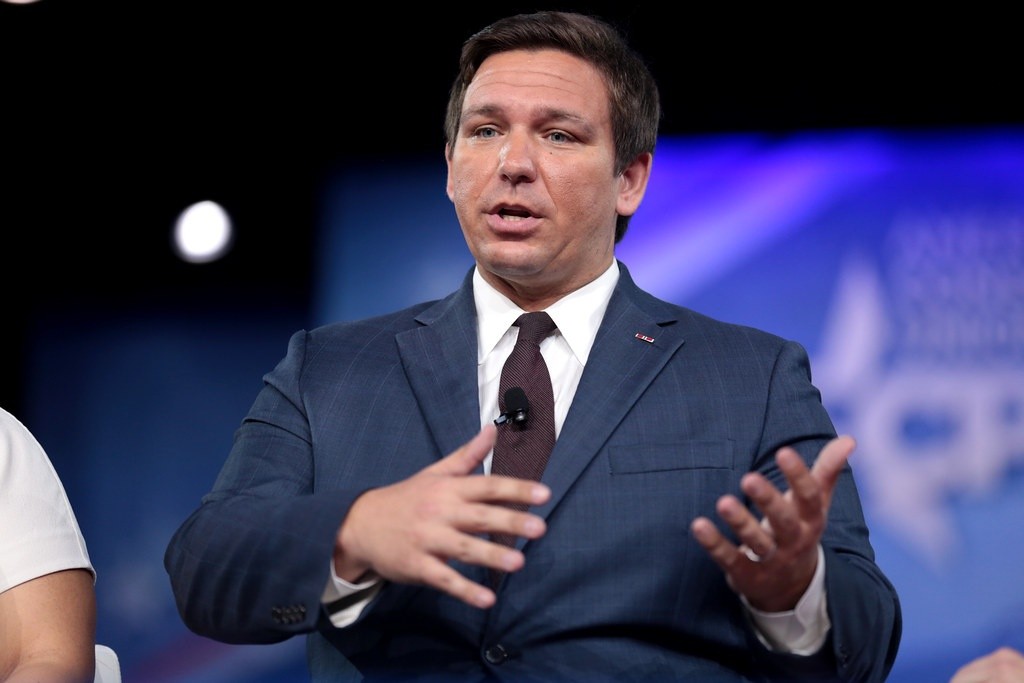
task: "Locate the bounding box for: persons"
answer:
[0,407,97,683]
[163,14,904,683]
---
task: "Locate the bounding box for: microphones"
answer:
[504,386,530,424]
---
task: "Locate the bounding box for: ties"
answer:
[490,311,559,584]
